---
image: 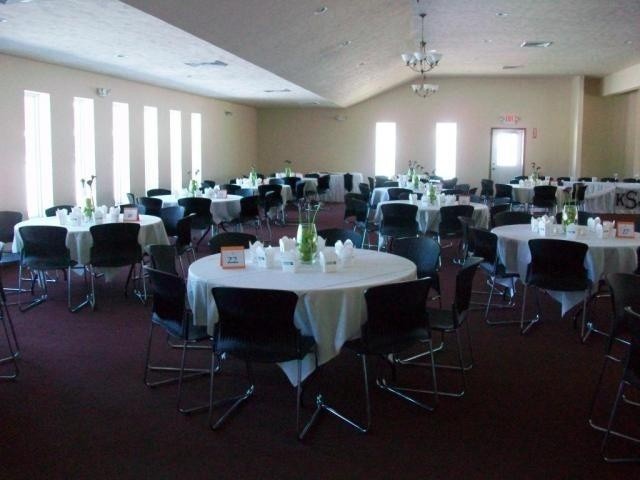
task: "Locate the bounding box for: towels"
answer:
[340,244,352,267]
[265,245,276,266]
[459,195,472,204]
[213,184,220,194]
[567,223,577,239]
[75,213,84,226]
[109,207,119,219]
[538,216,551,235]
[279,235,296,254]
[335,239,353,256]
[72,205,80,219]
[410,194,417,203]
[57,209,68,223]
[236,178,243,184]
[95,211,102,222]
[603,221,615,226]
[531,216,540,232]
[316,236,326,251]
[208,186,214,198]
[518,178,526,188]
[194,189,202,198]
[447,194,456,203]
[422,195,429,205]
[281,248,300,271]
[319,251,338,272]
[257,178,262,187]
[587,217,600,232]
[99,205,106,218]
[596,224,602,241]
[549,215,555,232]
[249,242,264,262]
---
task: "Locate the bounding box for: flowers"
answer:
[528,161,542,172]
[426,171,438,187]
[407,160,417,169]
[183,168,201,181]
[295,196,323,222]
[248,163,257,170]
[283,160,294,168]
[80,175,97,198]
[414,164,424,176]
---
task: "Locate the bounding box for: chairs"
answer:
[317,276,438,433]
[623,178,636,182]
[588,272,640,469]
[515,176,529,181]
[469,187,478,194]
[480,179,493,196]
[376,176,388,186]
[389,189,411,200]
[147,189,171,197]
[316,175,330,193]
[257,184,285,221]
[145,245,180,276]
[520,238,596,342]
[600,178,617,182]
[45,205,74,217]
[383,181,399,187]
[466,228,540,324]
[0,210,33,293]
[219,185,243,196]
[285,177,299,197]
[510,179,518,184]
[343,173,354,192]
[442,177,457,188]
[139,197,163,214]
[269,177,288,187]
[221,195,259,232]
[489,202,511,227]
[539,177,553,180]
[160,206,185,234]
[572,183,586,200]
[532,186,556,207]
[437,205,474,248]
[85,222,147,310]
[557,211,596,226]
[389,236,444,363]
[360,183,369,201]
[444,190,455,195]
[117,204,135,214]
[178,198,218,229]
[304,175,319,178]
[494,210,531,227]
[453,184,469,194]
[495,183,513,201]
[342,193,369,225]
[125,193,137,205]
[141,264,254,414]
[208,286,320,442]
[18,226,89,313]
[203,181,217,190]
[295,181,307,196]
[388,255,483,372]
[578,177,592,182]
[399,192,423,200]
[367,176,375,194]
[378,204,421,238]
[142,216,197,277]
[557,176,570,186]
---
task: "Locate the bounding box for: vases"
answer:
[249,172,258,186]
[426,186,438,204]
[531,173,539,181]
[82,198,96,221]
[295,223,318,263]
[407,168,413,180]
[284,168,289,176]
[188,180,198,194]
[562,206,580,232]
[412,173,419,188]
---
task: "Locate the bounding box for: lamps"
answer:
[401,14,442,74]
[411,74,440,99]
